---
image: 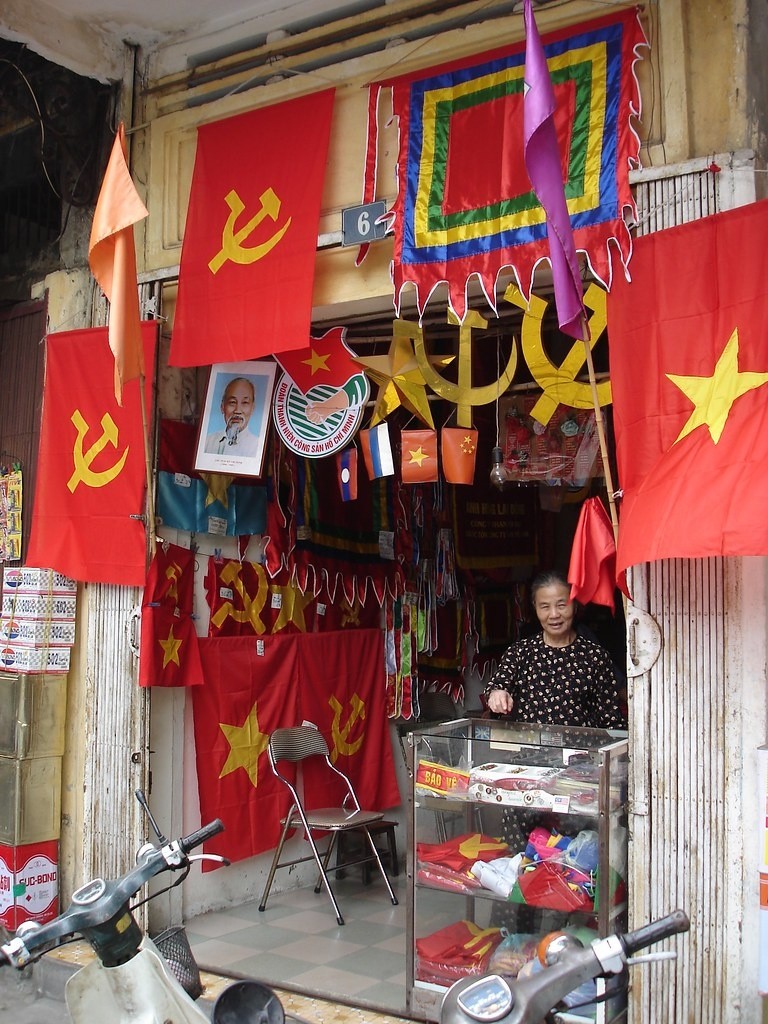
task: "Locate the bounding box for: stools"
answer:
[336,820,400,885]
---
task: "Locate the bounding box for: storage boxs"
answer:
[0,645,70,674]
[2,591,77,621]
[1,671,69,761]
[0,757,62,847]
[2,566,77,596]
[0,840,61,932]
[0,621,75,647]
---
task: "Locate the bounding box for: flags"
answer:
[138,417,541,872]
[523,0,591,343]
[441,429,478,485]
[336,448,357,502]
[359,422,394,480]
[24,319,157,586]
[87,122,150,408]
[401,430,438,483]
[605,199,768,602]
[169,84,334,367]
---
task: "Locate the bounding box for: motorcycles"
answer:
[0,788,231,1024]
[214,907,690,1024]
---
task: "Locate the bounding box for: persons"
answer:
[204,377,259,458]
[483,571,627,934]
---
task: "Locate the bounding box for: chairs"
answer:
[258,726,399,925]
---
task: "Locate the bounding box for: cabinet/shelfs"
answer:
[404,720,627,1024]
